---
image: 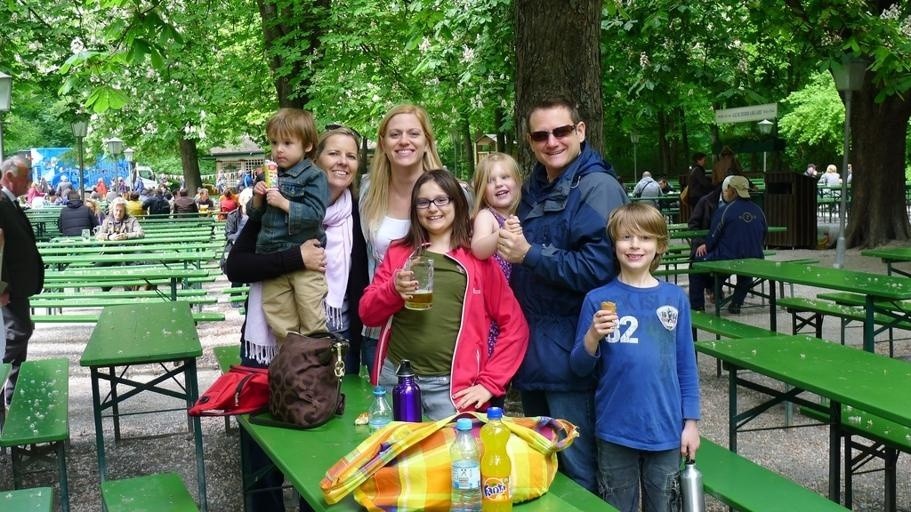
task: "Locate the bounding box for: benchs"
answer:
[0,357,71,511]
[0,487,55,512]
[680,435,853,512]
[816,291,910,345]
[661,208,681,211]
[763,249,775,256]
[99,471,200,512]
[0,362,12,392]
[798,402,910,512]
[652,244,690,283]
[661,212,680,223]
[690,309,795,433]
[213,344,240,438]
[774,297,911,339]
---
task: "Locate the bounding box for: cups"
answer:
[404,256,434,312]
[82,228,91,242]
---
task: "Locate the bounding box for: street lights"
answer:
[106,137,122,194]
[830,51,868,271]
[122,147,135,198]
[758,118,774,176]
[69,112,90,205]
[630,129,642,183]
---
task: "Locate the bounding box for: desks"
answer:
[24,201,250,324]
[623,179,680,207]
[691,258,911,379]
[749,177,765,190]
[78,299,208,512]
[236,373,623,512]
[665,222,688,229]
[860,246,911,358]
[693,432,911,512]
[817,183,852,222]
[690,258,911,352]
[667,229,711,243]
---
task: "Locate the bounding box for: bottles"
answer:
[392,359,423,423]
[480,407,513,511]
[368,386,393,437]
[450,418,480,512]
[680,459,705,512]
[93,226,105,243]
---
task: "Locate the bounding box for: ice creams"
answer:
[601,302,615,337]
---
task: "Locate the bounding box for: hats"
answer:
[729,176,750,198]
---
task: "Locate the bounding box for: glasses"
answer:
[530,125,575,141]
[416,197,452,209]
[325,124,362,138]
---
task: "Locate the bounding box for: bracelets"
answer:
[254,196,262,199]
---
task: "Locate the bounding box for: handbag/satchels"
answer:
[320,412,580,512]
[636,193,641,198]
[680,185,689,204]
[187,365,269,416]
[269,332,351,429]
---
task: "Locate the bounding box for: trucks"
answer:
[41,145,157,195]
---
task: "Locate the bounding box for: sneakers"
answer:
[728,303,740,314]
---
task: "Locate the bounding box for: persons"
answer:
[45,169,252,240]
[633,171,664,210]
[816,164,842,212]
[616,176,629,196]
[687,153,717,212]
[570,205,702,511]
[24,177,45,209]
[494,94,631,498]
[1,154,45,409]
[358,169,531,423]
[658,177,678,208]
[689,176,768,314]
[846,163,852,183]
[687,175,738,304]
[713,145,759,191]
[227,128,370,512]
[468,151,525,411]
[247,109,346,416]
[804,164,818,177]
[357,105,473,381]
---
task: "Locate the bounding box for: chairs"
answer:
[775,259,819,297]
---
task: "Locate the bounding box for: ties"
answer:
[14,200,29,224]
